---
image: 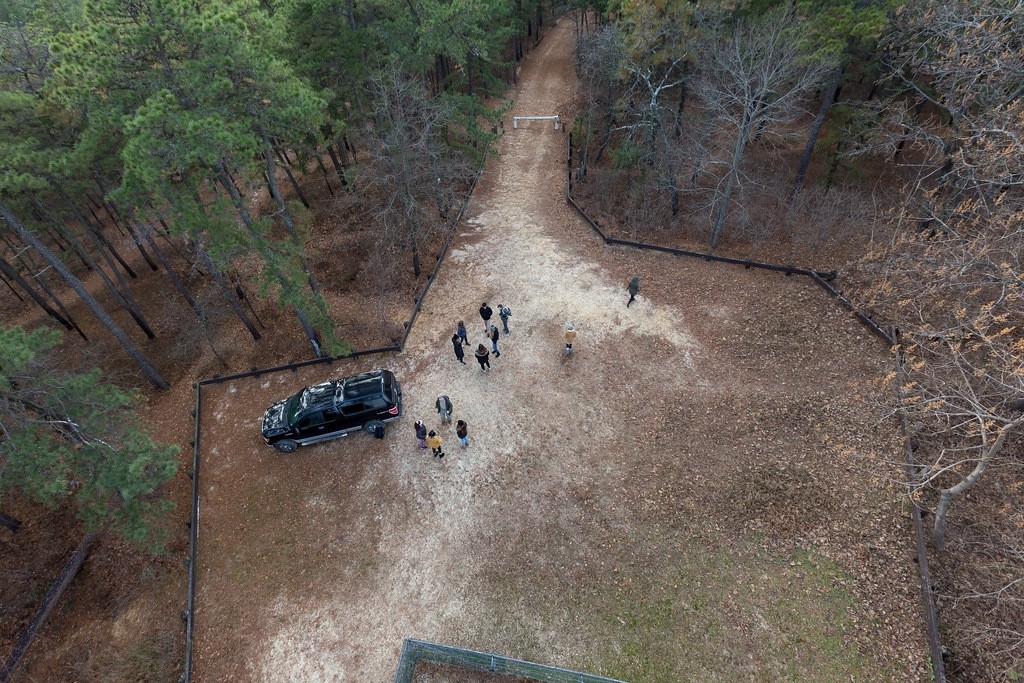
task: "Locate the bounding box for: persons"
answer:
[564,325,576,355]
[456,419,469,449]
[457,320,471,347]
[452,334,466,365]
[414,420,427,449]
[373,425,385,440]
[489,325,501,358]
[475,344,492,373]
[496,304,512,334]
[624,276,640,307]
[428,430,444,459]
[435,395,453,426]
[479,303,494,338]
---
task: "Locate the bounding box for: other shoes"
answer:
[434,451,438,457]
[421,446,428,448]
[466,343,471,345]
[503,329,509,334]
[449,420,452,424]
[442,421,445,425]
[566,348,572,354]
[492,350,496,353]
[487,334,489,338]
[481,368,486,373]
[488,368,490,369]
[457,358,461,360]
[461,362,466,364]
[461,446,466,449]
[485,329,487,332]
[465,443,469,446]
[496,352,500,357]
[439,453,444,458]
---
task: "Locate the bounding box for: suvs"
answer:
[257,369,402,453]
